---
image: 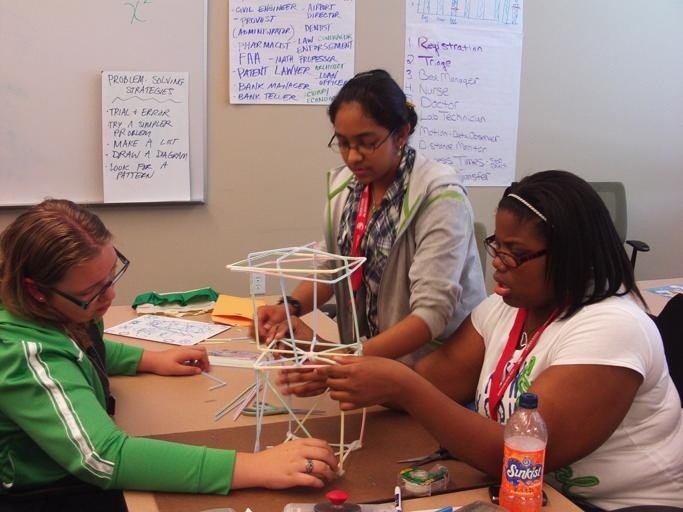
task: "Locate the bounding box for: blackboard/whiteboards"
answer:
[0,0,209,209]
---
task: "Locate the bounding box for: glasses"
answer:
[36,246,130,311]
[327,126,397,156]
[483,233,545,269]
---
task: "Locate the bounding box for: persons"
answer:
[309,166,683,511]
[248,66,491,412]
[1,196,341,511]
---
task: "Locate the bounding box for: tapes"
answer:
[393,466,450,494]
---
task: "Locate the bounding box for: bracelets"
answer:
[344,347,355,354]
[275,293,302,319]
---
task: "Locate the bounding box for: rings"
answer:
[304,457,314,474]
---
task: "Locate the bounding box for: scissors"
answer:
[242,400,323,417]
[397,448,452,466]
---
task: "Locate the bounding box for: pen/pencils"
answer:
[434,506,452,512]
[392,484,402,510]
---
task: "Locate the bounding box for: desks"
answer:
[100,293,584,512]
[618,277,682,317]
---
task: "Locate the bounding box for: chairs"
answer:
[473,221,488,283]
[587,181,649,270]
[652,294,683,409]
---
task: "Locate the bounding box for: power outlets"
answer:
[249,270,266,296]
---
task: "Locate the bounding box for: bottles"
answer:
[496,393,549,512]
[313,491,361,512]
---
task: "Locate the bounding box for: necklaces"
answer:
[519,313,550,349]
[367,184,380,214]
